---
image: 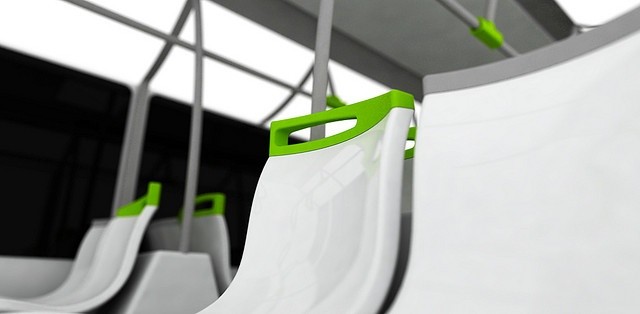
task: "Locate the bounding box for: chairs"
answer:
[191,91,414,313]
[386,6,640,314]
[400,125,415,219]
[0,183,161,312]
[179,194,237,290]
